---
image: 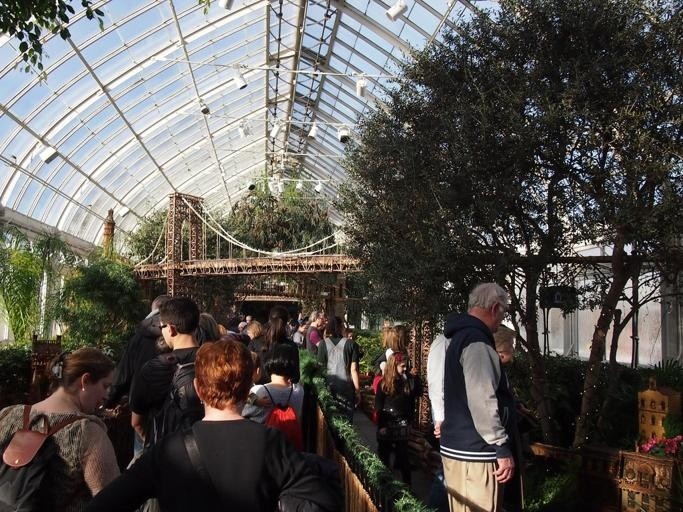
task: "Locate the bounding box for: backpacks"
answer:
[147,353,204,440]
[0,405,87,512]
[263,384,303,453]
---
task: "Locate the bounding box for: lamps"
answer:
[219,1,234,12]
[356,80,367,96]
[233,72,247,90]
[270,124,280,140]
[201,103,211,116]
[248,181,256,191]
[308,126,319,141]
[384,2,409,23]
[315,184,323,193]
[338,126,351,143]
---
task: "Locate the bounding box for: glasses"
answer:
[159,325,167,329]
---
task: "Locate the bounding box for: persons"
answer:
[318,316,362,426]
[374,324,425,472]
[438,281,519,512]
[494,323,520,512]
[0,293,332,511]
[427,319,454,439]
